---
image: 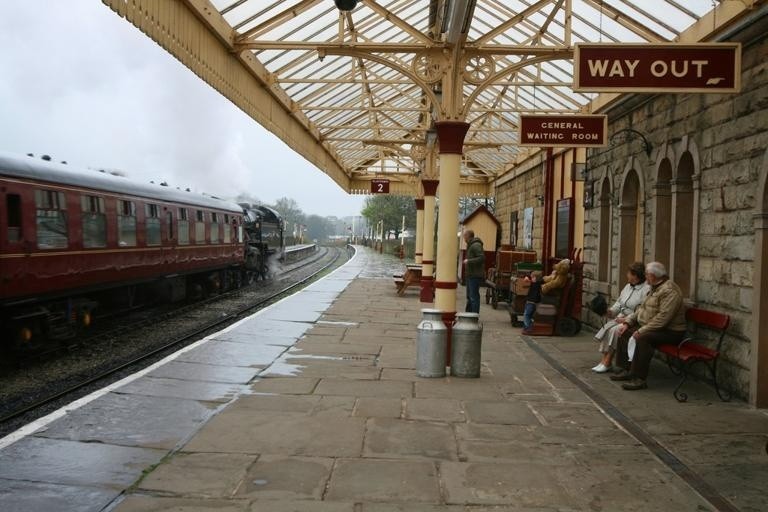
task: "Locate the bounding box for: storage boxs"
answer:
[497,251,537,272]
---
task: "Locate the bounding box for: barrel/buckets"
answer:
[449,312,484,378]
[417,310,448,377]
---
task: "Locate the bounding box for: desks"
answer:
[397,265,422,296]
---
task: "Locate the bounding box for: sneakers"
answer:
[622,379,648,390]
[609,370,633,381]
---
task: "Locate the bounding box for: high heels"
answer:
[591,362,603,372]
[596,364,613,373]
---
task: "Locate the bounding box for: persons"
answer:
[610,261,689,384]
[460,228,488,322]
[522,270,546,331]
[591,264,655,372]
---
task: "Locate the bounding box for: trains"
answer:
[0,151,284,351]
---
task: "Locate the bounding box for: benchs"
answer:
[653,309,733,402]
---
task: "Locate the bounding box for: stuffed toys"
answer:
[543,258,571,293]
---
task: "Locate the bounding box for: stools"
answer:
[394,278,404,283]
[393,273,401,276]
